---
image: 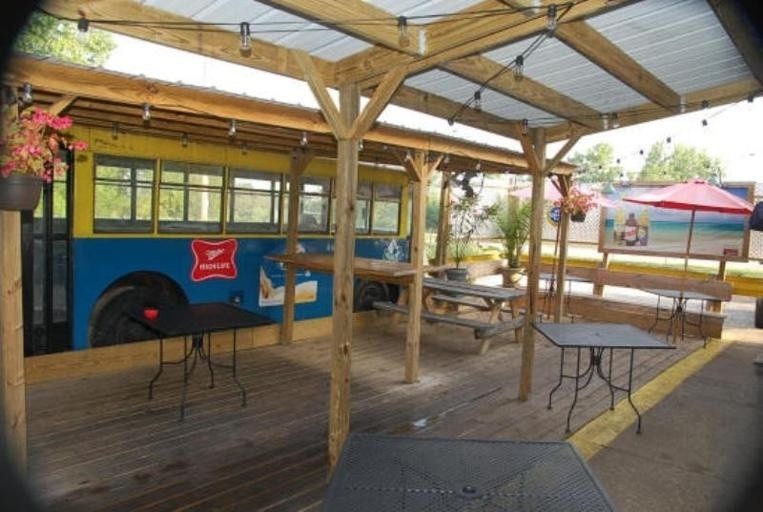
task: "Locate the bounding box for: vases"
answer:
[569,211,586,222]
[0,173,45,212]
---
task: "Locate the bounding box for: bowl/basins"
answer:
[144,309,159,321]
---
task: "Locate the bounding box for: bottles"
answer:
[613,207,649,247]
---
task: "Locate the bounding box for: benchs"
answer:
[371,301,493,336]
[430,294,526,319]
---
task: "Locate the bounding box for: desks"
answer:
[646,289,722,349]
[323,432,613,509]
[532,323,677,435]
[389,279,530,355]
[126,302,276,421]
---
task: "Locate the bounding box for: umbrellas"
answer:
[622,177,754,273]
[508,177,620,275]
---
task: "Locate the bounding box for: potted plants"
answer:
[440,193,532,288]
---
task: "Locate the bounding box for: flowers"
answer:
[551,188,598,216]
[0,106,88,184]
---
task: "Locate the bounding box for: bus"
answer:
[21,124,417,355]
